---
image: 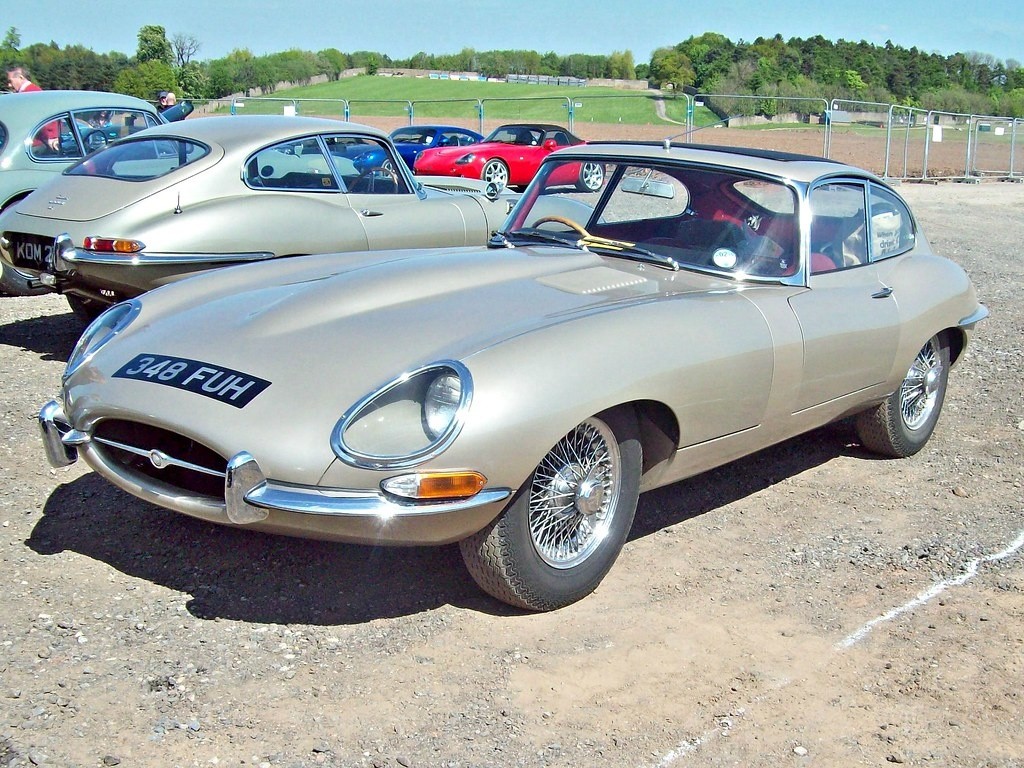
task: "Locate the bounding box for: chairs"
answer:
[553,133,570,145]
[514,131,537,144]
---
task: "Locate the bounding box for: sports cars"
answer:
[1,88,607,299]
[36,137,992,613]
[1,113,608,329]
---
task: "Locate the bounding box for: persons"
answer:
[156,91,186,122]
[89,111,114,129]
[6,65,62,155]
